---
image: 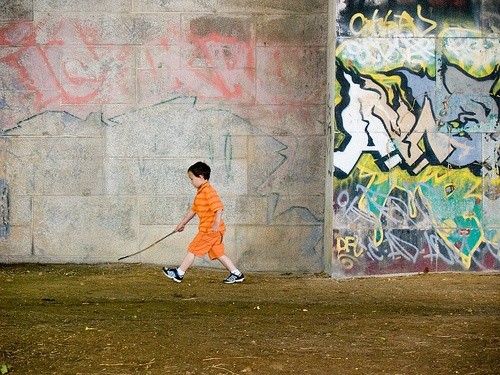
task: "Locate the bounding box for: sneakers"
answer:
[223,271,245,283]
[161,267,183,283]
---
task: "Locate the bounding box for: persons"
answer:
[162,161,244,285]
